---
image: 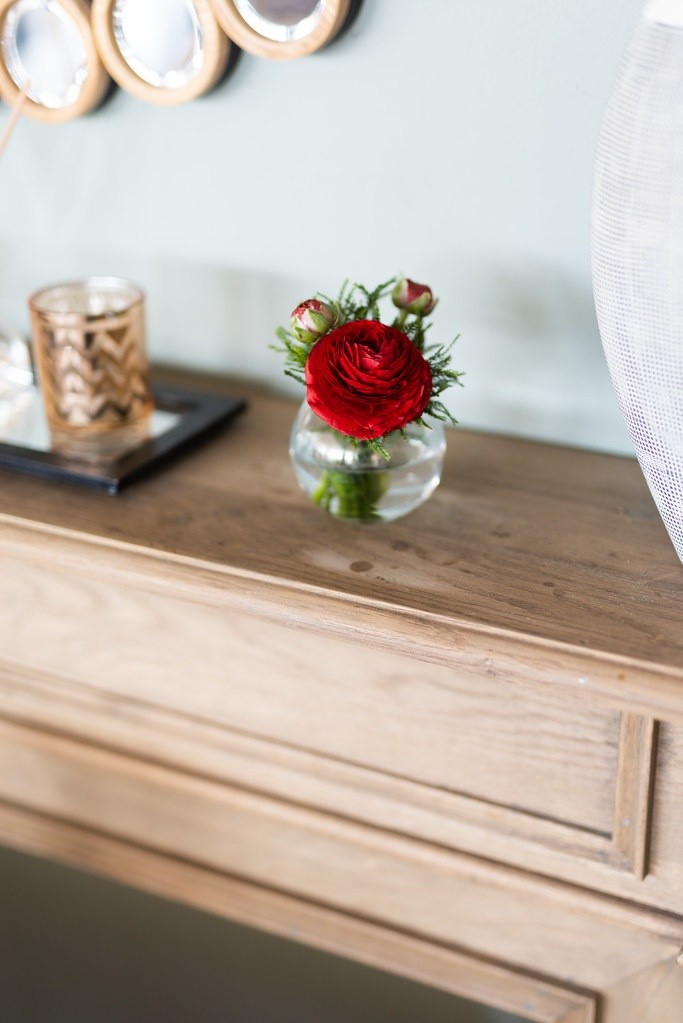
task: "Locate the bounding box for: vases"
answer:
[290,396,449,524]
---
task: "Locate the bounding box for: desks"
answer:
[1,354,683,1023]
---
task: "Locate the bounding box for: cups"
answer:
[28,277,154,451]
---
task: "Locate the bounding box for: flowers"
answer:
[268,276,467,524]
